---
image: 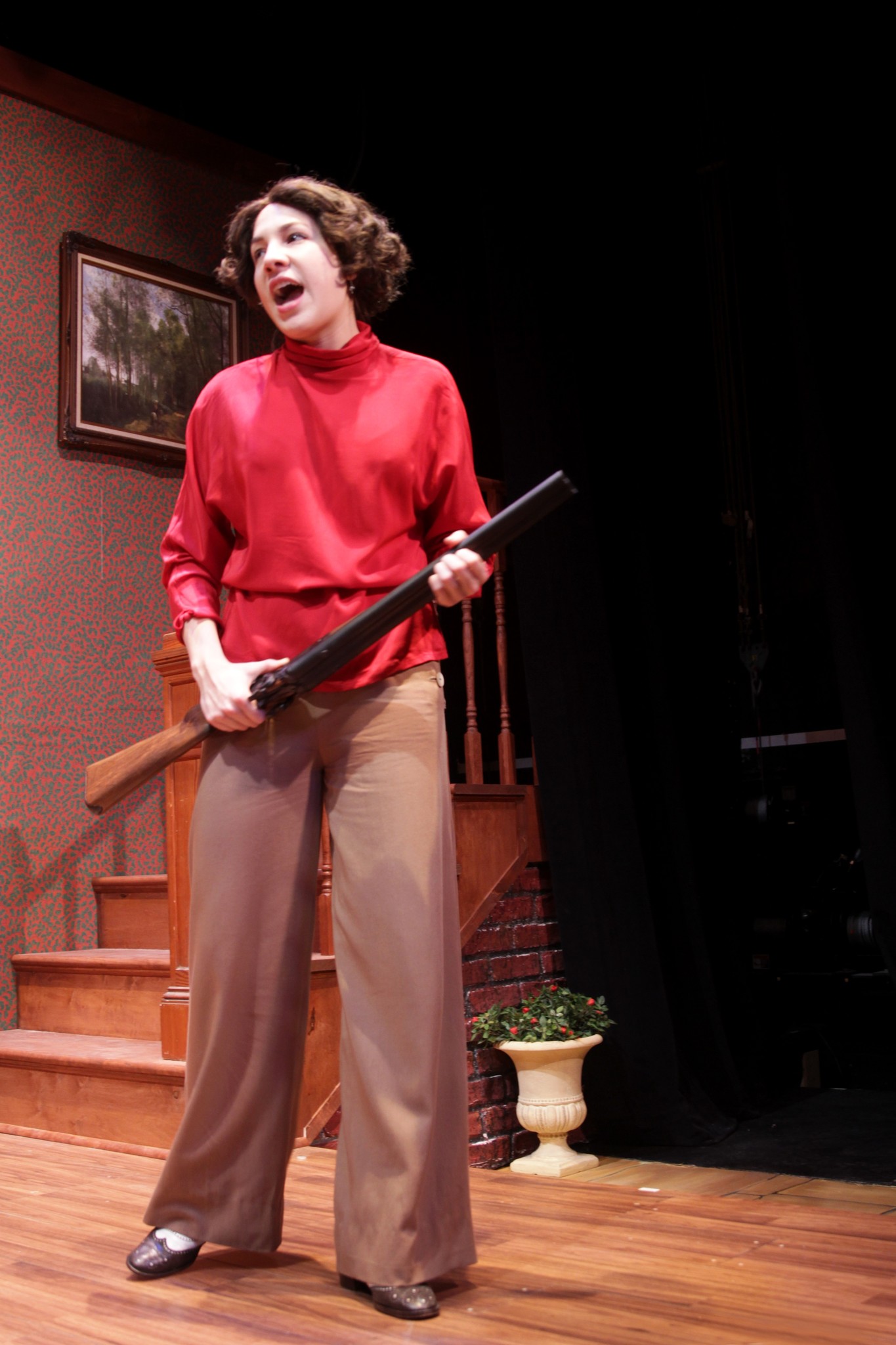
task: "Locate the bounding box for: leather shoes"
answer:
[337,1272,440,1321]
[127,1227,207,1280]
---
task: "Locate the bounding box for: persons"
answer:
[126,178,494,1321]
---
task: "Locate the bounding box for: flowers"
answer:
[469,983,615,1049]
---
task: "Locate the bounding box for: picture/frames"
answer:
[58,230,249,465]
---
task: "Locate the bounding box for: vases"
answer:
[493,1034,603,1178]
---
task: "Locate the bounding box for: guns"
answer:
[85,471,569,818]
